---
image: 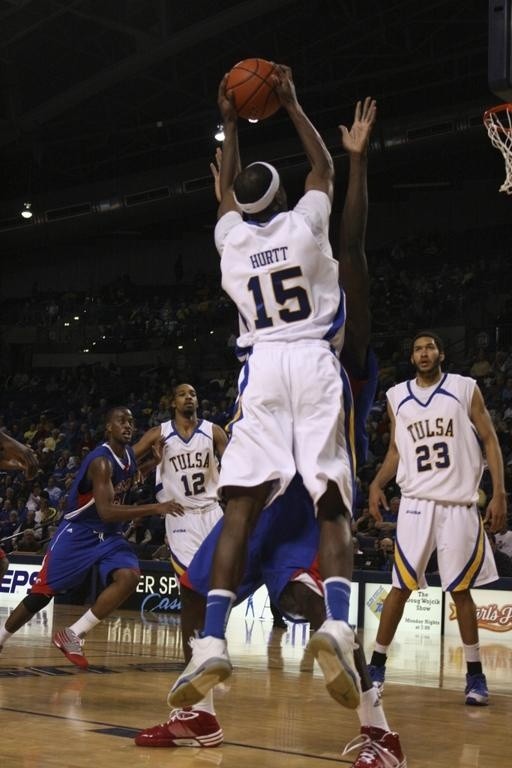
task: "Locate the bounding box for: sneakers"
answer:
[464,673,490,706]
[135,703,222,747]
[267,620,385,709]
[52,626,88,668]
[167,636,235,710]
[341,727,406,768]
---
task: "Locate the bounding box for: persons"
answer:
[130,379,230,662]
[366,329,509,706]
[2,243,511,627]
[163,62,366,713]
[2,400,186,672]
[130,93,413,768]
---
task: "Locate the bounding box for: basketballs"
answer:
[224,58,282,119]
